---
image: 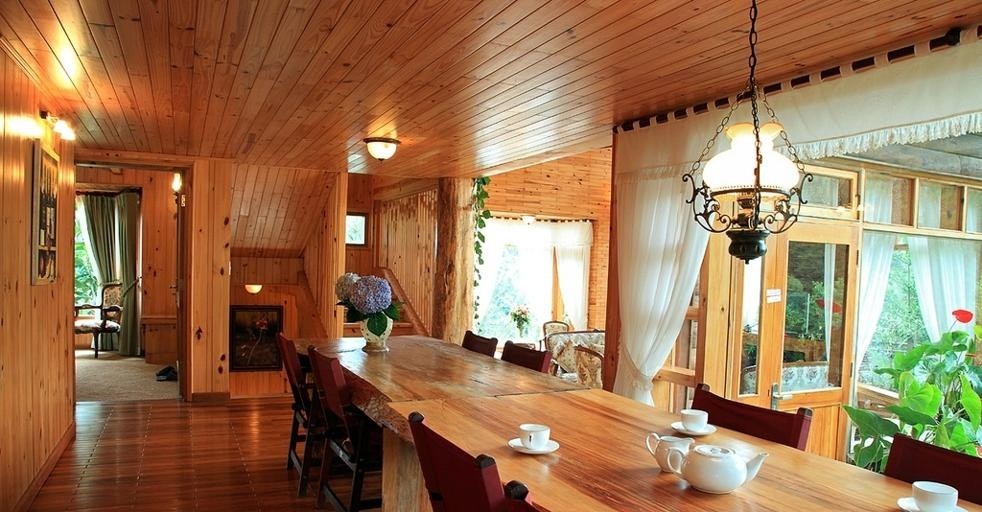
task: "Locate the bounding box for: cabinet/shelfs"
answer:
[176,161,231,402]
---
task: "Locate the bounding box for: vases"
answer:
[359,314,394,353]
[520,326,526,337]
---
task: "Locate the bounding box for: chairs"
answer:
[884,432,982,506]
[500,341,553,374]
[462,330,498,358]
[503,479,531,512]
[554,345,604,389]
[546,329,606,352]
[75,282,123,359]
[690,383,813,452]
[543,321,569,336]
[306,346,381,512]
[407,411,503,512]
[275,331,326,499]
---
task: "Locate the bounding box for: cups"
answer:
[912,481,958,512]
[519,423,550,450]
[680,409,708,432]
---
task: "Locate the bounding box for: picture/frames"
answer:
[31,137,61,287]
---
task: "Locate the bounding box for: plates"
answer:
[896,497,969,512]
[507,437,560,454]
[671,421,717,435]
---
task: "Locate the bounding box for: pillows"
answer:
[556,340,577,373]
[589,342,605,355]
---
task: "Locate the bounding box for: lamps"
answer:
[244,285,263,294]
[682,0,814,265]
[41,111,76,142]
[363,138,402,165]
[171,172,182,193]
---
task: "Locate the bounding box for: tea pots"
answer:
[666,444,770,494]
[645,432,696,473]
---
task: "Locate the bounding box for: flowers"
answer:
[512,305,530,329]
[333,271,407,339]
